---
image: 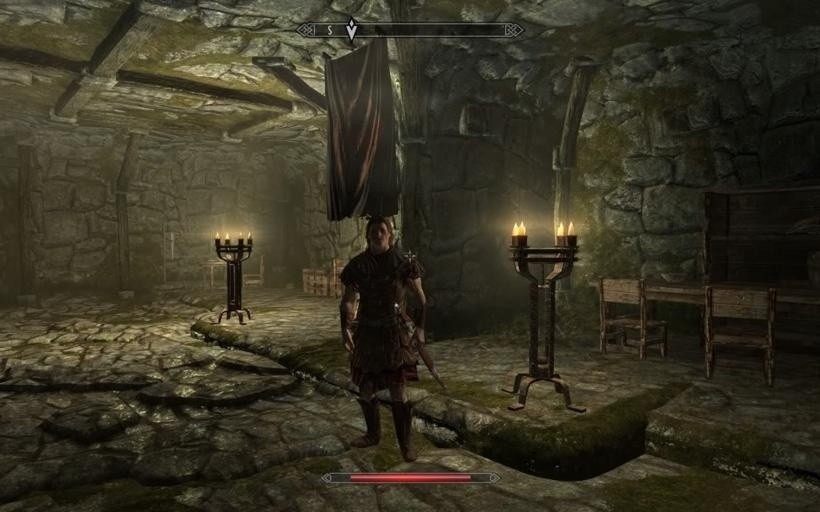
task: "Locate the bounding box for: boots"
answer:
[351,395,381,448]
[390,400,417,462]
